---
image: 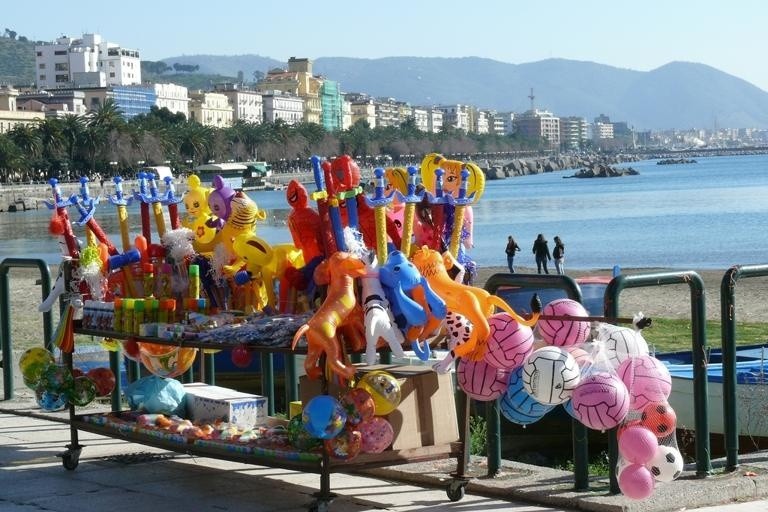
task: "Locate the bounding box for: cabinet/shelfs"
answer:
[53,289,473,511]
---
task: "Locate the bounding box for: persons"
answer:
[504,236,521,273]
[553,235,566,275]
[532,233,552,275]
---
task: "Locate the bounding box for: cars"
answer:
[494,277,615,353]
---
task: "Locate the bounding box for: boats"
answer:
[189,161,272,196]
